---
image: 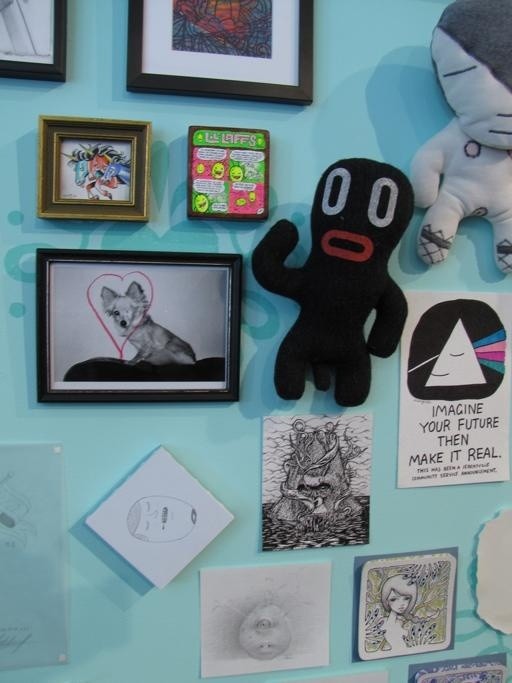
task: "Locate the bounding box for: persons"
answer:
[378,573,440,646]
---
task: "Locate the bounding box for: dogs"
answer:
[101,281,196,367]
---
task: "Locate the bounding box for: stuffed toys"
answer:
[407,0,512,273]
[252,156,415,406]
